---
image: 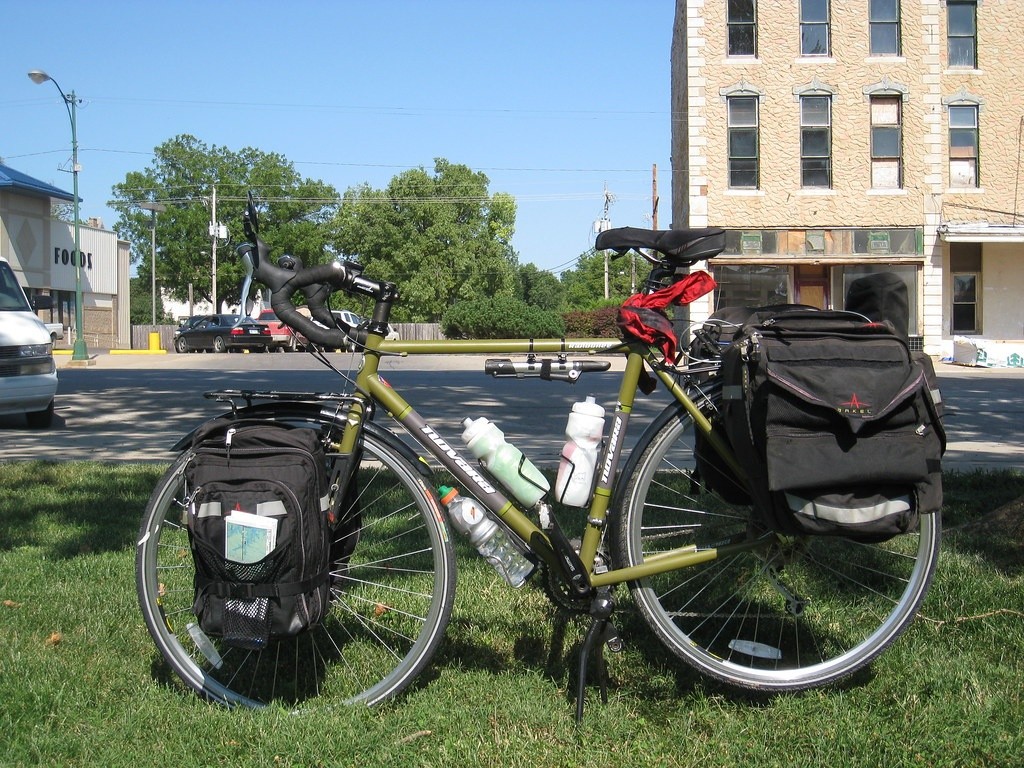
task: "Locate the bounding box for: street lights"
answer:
[25,68,89,359]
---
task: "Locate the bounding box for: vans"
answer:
[0,255,58,429]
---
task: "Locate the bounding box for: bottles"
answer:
[437,485,534,586]
[555,394,605,508]
[460,415,550,509]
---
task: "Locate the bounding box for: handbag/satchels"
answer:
[721,309,948,545]
[689,307,928,524]
[186,417,334,654]
[272,450,363,601]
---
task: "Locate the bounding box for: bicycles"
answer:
[132,188,945,752]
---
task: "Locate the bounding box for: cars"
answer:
[251,307,400,354]
[172,314,272,353]
